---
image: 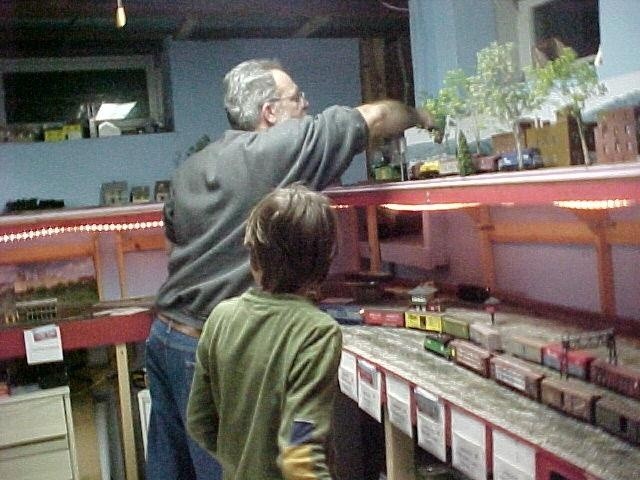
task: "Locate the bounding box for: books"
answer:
[322,291,640,445]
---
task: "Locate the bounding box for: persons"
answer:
[186,181,345,480]
[140,59,437,480]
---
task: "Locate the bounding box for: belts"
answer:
[156,312,201,339]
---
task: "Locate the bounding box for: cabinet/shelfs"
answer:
[0,160,640,480]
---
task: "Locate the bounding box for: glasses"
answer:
[268,91,305,104]
